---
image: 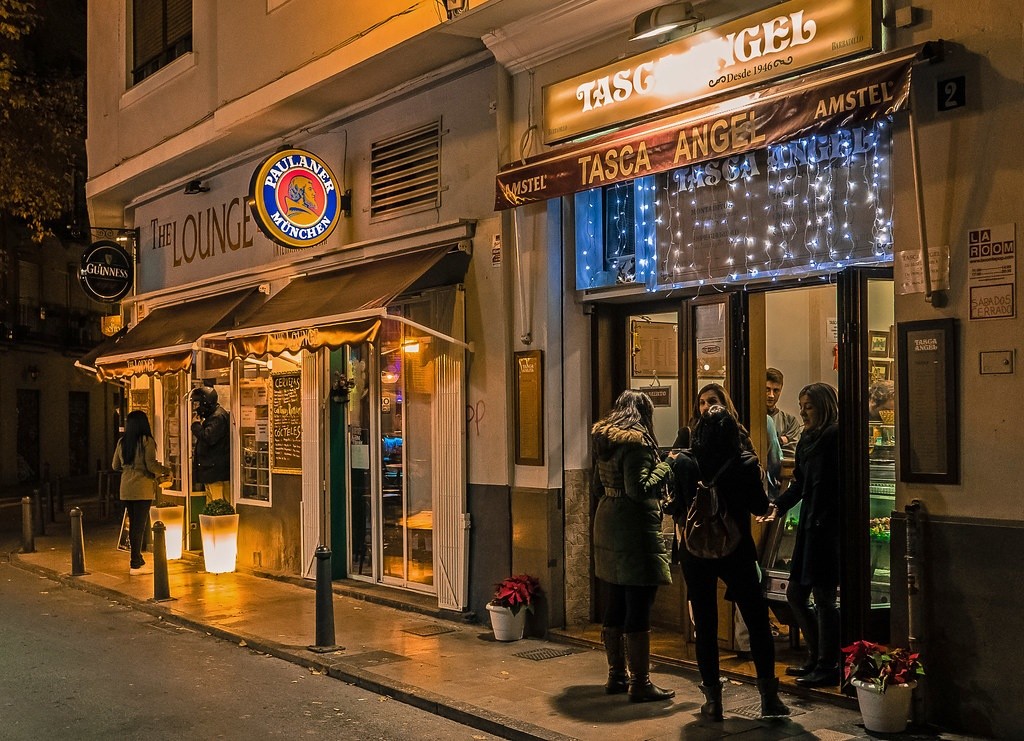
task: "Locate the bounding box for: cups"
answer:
[672,449,683,454]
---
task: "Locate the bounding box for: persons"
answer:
[190,387,230,556]
[668,383,792,722]
[765,368,799,446]
[756,383,841,685]
[870,380,895,421]
[112,410,172,576]
[723,380,783,497]
[589,390,681,702]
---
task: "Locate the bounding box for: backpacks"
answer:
[677,449,742,558]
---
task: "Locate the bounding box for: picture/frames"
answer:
[868,324,894,388]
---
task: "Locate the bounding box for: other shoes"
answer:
[129,565,153,575]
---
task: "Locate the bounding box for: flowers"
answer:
[840,639,926,695]
[331,371,355,398]
[490,574,539,617]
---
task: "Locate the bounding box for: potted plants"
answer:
[199,498,239,573]
[149,501,185,560]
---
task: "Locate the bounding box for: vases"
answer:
[485,602,528,640]
[850,676,918,732]
[334,395,350,404]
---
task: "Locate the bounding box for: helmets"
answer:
[189,386,219,416]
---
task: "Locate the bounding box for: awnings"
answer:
[97,286,274,382]
[76,339,131,389]
[493,37,961,344]
[228,243,473,366]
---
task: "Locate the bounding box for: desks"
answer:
[386,464,403,489]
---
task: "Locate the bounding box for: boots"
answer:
[624,630,676,703]
[758,676,791,715]
[600,626,632,693]
[698,680,724,721]
[785,648,819,677]
[794,658,841,685]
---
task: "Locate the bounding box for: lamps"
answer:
[275,144,294,152]
[627,1,705,43]
[115,229,136,242]
[183,180,211,194]
